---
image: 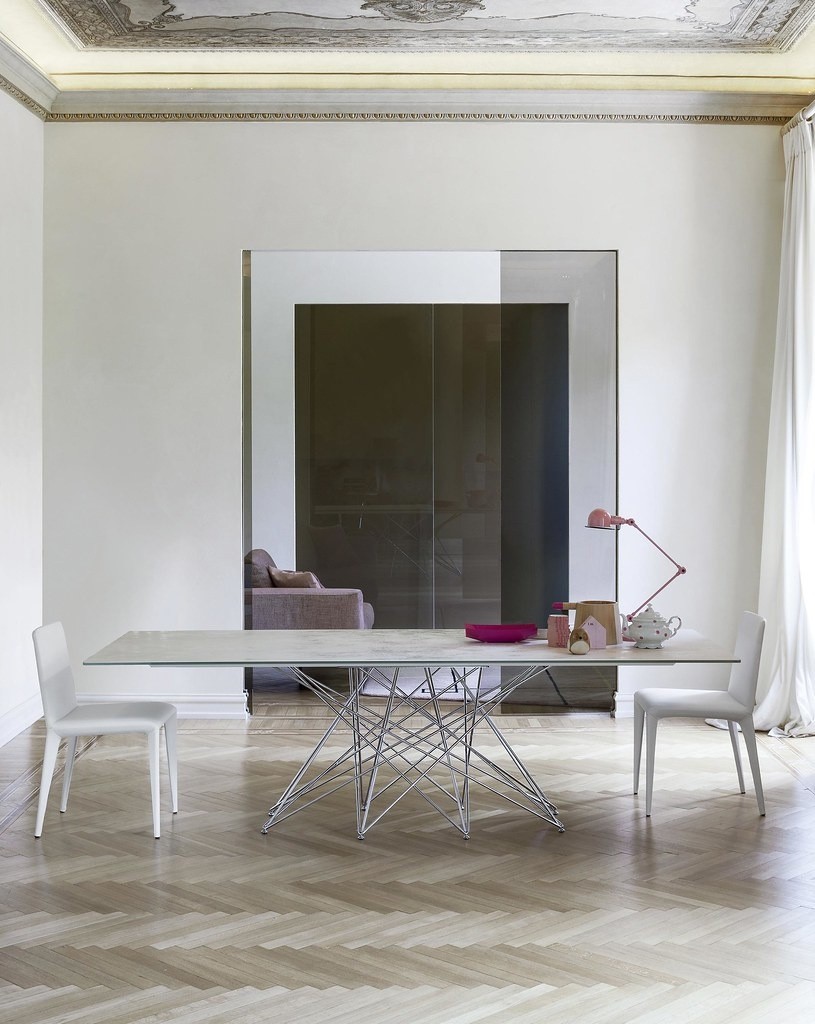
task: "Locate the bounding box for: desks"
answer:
[314,500,502,582]
[83,629,741,840]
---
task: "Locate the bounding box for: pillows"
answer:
[268,565,321,588]
[305,526,355,568]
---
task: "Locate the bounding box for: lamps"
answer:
[475,453,501,507]
[585,508,687,642]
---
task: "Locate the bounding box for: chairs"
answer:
[633,610,767,820]
[32,620,179,840]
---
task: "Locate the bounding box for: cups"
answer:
[547,615,569,648]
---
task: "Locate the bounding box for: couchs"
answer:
[243,549,374,690]
[307,526,380,608]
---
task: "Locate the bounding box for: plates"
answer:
[465,624,537,642]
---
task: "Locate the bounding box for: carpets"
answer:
[362,666,617,709]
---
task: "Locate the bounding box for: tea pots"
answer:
[619,604,681,648]
